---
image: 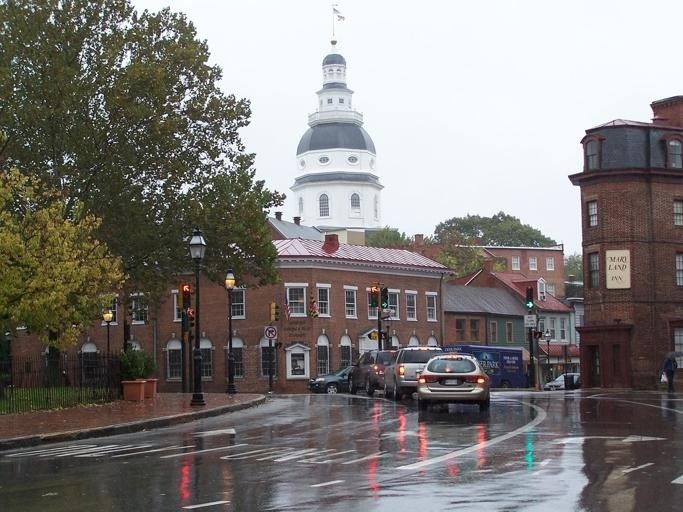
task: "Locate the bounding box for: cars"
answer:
[308,347,490,408]
[543,372,581,390]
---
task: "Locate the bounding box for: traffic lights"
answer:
[532,329,542,340]
[269,302,280,322]
[186,309,195,327]
[527,286,533,311]
[370,285,389,309]
[182,285,190,308]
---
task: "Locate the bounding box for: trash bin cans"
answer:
[564,375,574,390]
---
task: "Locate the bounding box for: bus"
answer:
[442,344,524,388]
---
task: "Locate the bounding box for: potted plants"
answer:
[118,346,158,402]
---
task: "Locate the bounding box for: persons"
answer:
[661,359,678,393]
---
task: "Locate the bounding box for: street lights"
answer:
[189,226,207,406]
[225,268,236,395]
[544,328,551,382]
[103,307,113,401]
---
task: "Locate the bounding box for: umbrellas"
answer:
[666,352,683,359]
